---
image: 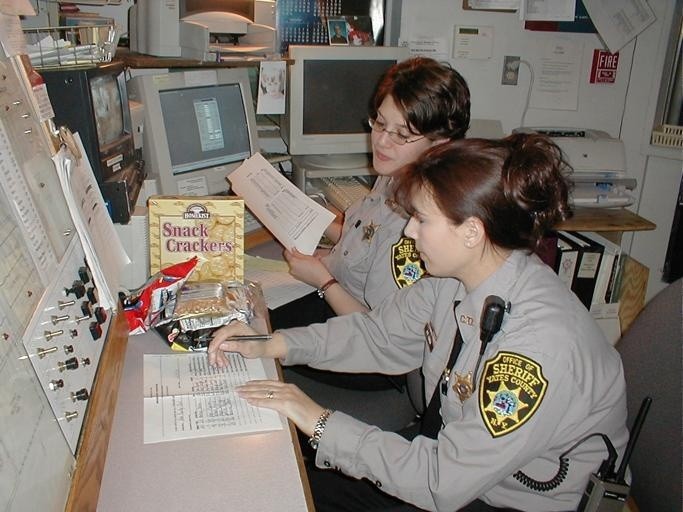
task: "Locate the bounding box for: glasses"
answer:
[365,109,427,147]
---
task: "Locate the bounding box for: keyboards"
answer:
[310,176,372,214]
[244,206,263,236]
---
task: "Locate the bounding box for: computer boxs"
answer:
[100,147,146,226]
[290,156,381,198]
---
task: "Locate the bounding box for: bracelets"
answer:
[317,279,339,299]
[307,407,334,450]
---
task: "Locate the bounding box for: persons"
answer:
[207,132,633,512]
[267,55,471,392]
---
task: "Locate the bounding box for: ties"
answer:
[418,303,464,437]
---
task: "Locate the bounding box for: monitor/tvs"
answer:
[33,62,136,184]
[126,66,262,197]
[280,44,412,168]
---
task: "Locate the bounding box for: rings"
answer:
[264,391,274,399]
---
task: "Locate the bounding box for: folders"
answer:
[553,231,605,312]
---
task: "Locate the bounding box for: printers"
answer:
[512,126,637,210]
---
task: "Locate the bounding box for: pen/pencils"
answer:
[193,335,273,341]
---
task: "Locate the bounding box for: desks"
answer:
[63,275,319,512]
[545,207,654,241]
